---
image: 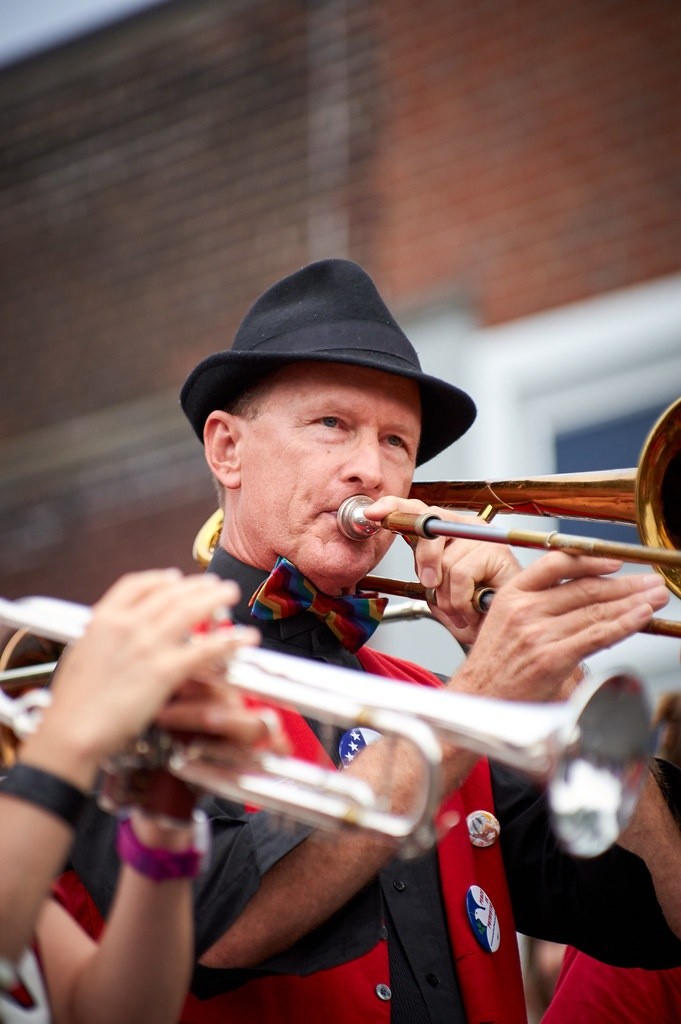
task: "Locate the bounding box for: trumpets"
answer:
[0,591,659,859]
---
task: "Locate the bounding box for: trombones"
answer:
[189,393,681,646]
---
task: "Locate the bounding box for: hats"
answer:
[178,259,478,470]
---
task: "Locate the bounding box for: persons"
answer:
[0,258,681,1024]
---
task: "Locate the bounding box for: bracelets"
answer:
[0,764,88,832]
[116,819,202,880]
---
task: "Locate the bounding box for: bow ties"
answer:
[248,555,388,654]
